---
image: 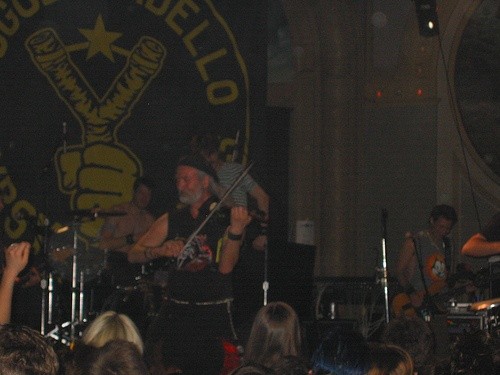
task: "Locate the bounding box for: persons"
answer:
[460,212,500,259]
[393,205,483,375]
[127,156,255,333]
[109,178,162,281]
[0,241,500,375]
[188,130,270,257]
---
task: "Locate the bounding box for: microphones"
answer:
[381,208,388,238]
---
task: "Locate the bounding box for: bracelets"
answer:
[227,232,244,241]
[126,234,133,243]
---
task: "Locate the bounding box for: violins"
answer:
[197,196,267,233]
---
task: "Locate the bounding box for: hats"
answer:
[176,152,220,183]
